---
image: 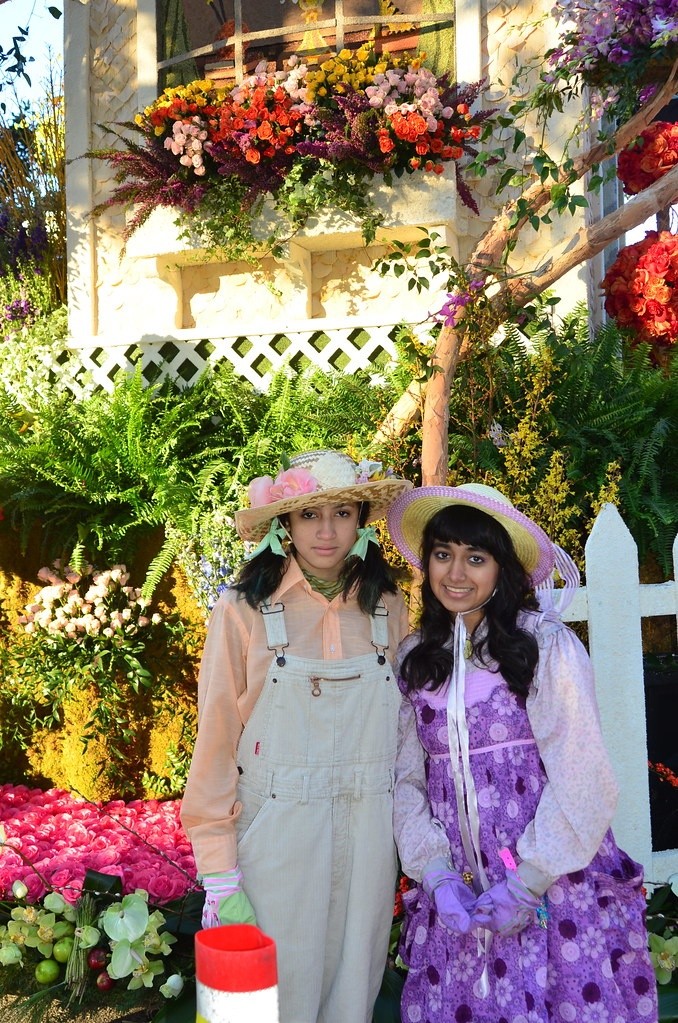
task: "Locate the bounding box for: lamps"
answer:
[82,868,123,904]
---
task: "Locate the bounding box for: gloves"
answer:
[422,871,476,935]
[473,868,544,938]
[201,864,257,929]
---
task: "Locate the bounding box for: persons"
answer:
[179,450,410,1023]
[388,483,657,1023]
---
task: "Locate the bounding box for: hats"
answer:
[234,451,413,542]
[387,483,581,615]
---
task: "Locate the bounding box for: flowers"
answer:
[463,0,678,374]
[248,461,382,507]
[64,43,506,294]
